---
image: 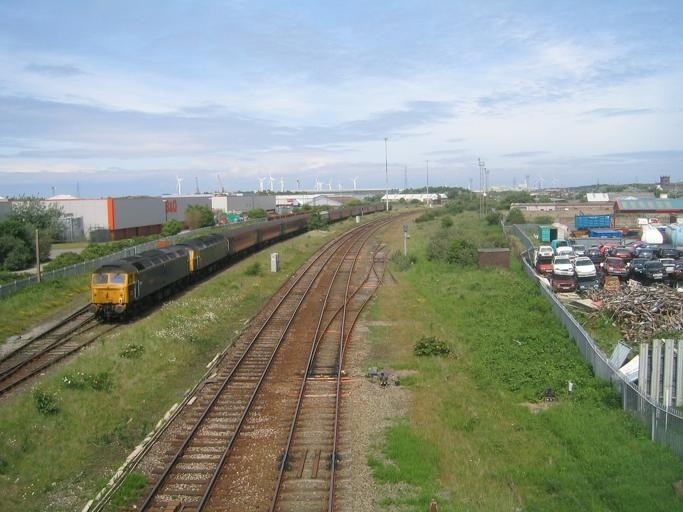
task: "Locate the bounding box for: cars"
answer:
[533,240,682,293]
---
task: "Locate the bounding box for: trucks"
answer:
[637,218,660,225]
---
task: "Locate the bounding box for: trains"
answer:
[89,202,392,324]
